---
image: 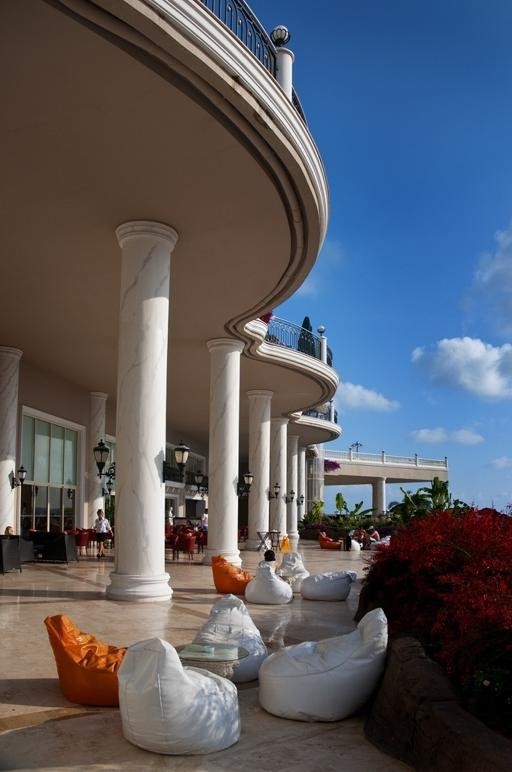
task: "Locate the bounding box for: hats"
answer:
[264,550,276,561]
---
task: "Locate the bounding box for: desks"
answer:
[256,531,281,552]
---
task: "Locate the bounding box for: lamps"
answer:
[194,469,208,493]
[268,482,303,505]
[93,439,115,494]
[67,489,72,498]
[162,440,191,483]
[237,470,254,497]
[12,465,27,489]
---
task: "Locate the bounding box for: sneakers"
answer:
[101,554,105,557]
[97,553,101,559]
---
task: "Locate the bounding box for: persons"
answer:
[186,520,193,528]
[259,549,279,574]
[169,507,175,526]
[201,509,209,532]
[93,508,114,559]
[346,528,359,551]
[4,526,13,535]
[323,531,336,542]
[362,525,380,550]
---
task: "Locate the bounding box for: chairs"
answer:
[29,528,98,576]
[165,523,208,561]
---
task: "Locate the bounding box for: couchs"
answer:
[211,553,253,594]
[0,530,79,576]
[193,594,268,684]
[116,636,241,756]
[300,570,357,602]
[244,557,294,604]
[275,551,310,593]
[258,608,388,724]
[44,614,128,707]
[319,531,391,551]
